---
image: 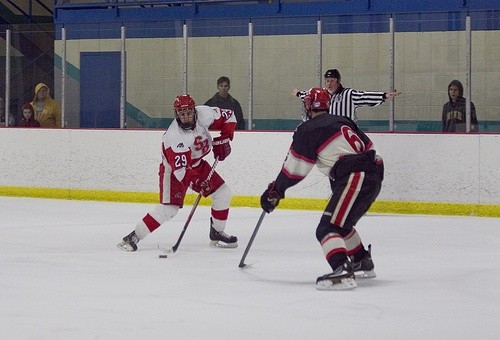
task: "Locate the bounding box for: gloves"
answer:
[260,180,281,213]
[212,136,231,161]
[188,174,214,197]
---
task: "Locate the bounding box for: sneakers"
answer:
[117,230,140,253]
[315,256,359,290]
[209,227,239,248]
[350,244,376,279]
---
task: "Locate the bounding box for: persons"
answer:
[292,69,400,127]
[261,87,384,289]
[117,94,238,253]
[205,76,246,131]
[442,79,478,132]
[0,83,60,128]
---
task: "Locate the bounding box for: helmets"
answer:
[173,94,198,130]
[301,87,331,122]
[324,69,341,79]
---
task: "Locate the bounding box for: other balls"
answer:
[158,255,167,258]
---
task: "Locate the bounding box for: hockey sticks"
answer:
[238,196,273,269]
[157,154,220,255]
